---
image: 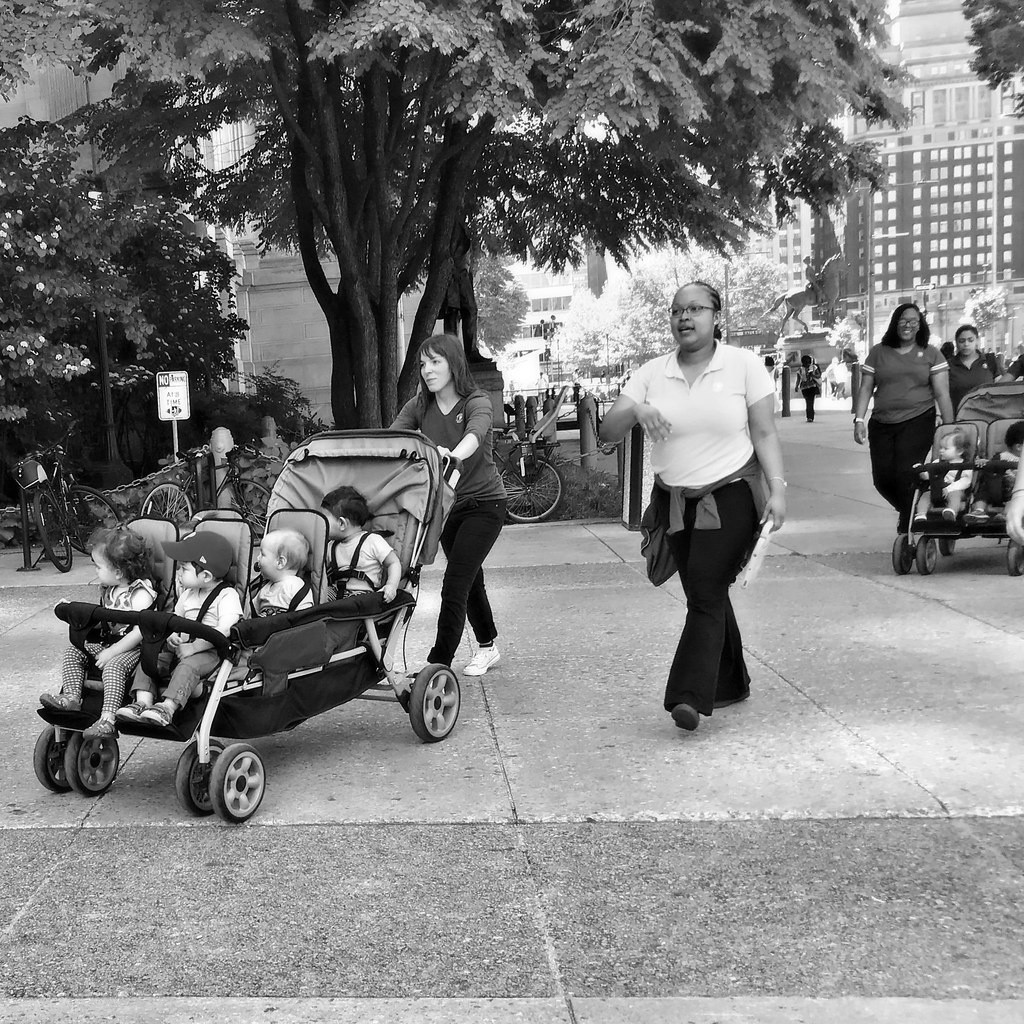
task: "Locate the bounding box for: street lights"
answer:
[951,262,1016,295]
[868,179,938,358]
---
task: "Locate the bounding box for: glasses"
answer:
[898,319,920,327]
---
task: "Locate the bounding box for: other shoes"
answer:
[942,508,957,522]
[807,418,813,422]
[914,515,928,523]
[671,703,700,731]
[714,684,750,708]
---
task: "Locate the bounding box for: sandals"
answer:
[83,717,119,740]
[40,694,83,711]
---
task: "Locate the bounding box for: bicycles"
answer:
[138,443,272,548]
[491,425,564,523]
[8,419,124,574]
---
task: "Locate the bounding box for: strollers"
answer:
[892,382,1024,576]
[32,427,468,826]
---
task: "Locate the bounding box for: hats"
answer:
[160,532,232,578]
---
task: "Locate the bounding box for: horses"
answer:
[758,252,851,337]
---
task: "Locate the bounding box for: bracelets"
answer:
[772,477,787,488]
[936,413,942,416]
[1011,489,1024,496]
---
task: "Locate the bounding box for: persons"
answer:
[853,301,956,535]
[535,371,549,406]
[912,426,972,524]
[509,380,515,403]
[115,530,244,726]
[795,355,821,421]
[39,526,157,741]
[825,356,851,402]
[235,525,313,668]
[763,354,781,413]
[626,368,632,381]
[963,420,1024,524]
[599,282,788,731]
[572,368,583,406]
[1006,443,1024,544]
[319,485,380,606]
[389,334,509,676]
[600,371,606,383]
[934,324,1024,430]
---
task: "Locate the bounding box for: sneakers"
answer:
[962,509,990,524]
[994,514,1006,521]
[116,701,146,723]
[141,702,173,726]
[463,644,502,676]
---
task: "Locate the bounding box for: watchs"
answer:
[854,418,864,423]
